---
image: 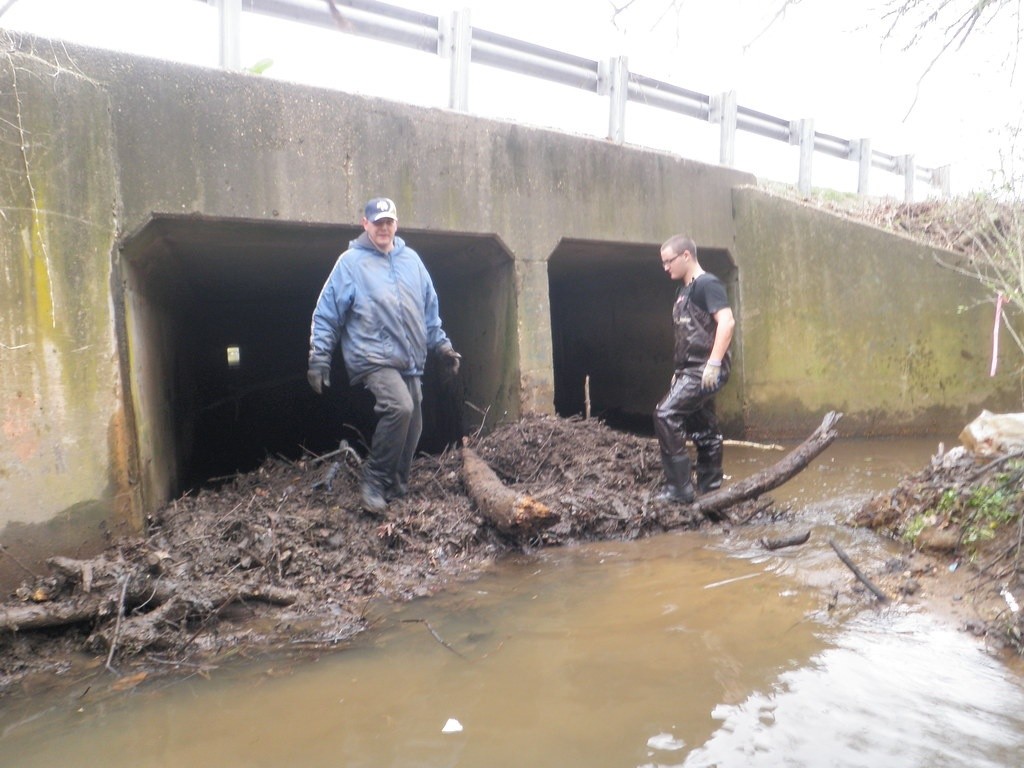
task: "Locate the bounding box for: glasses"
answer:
[662,253,683,266]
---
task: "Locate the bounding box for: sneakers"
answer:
[645,488,697,506]
[357,481,390,515]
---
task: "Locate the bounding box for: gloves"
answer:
[307,367,331,394]
[435,338,463,374]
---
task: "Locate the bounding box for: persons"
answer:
[650,232,740,509]
[305,197,463,511]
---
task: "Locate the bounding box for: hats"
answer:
[364,196,398,223]
